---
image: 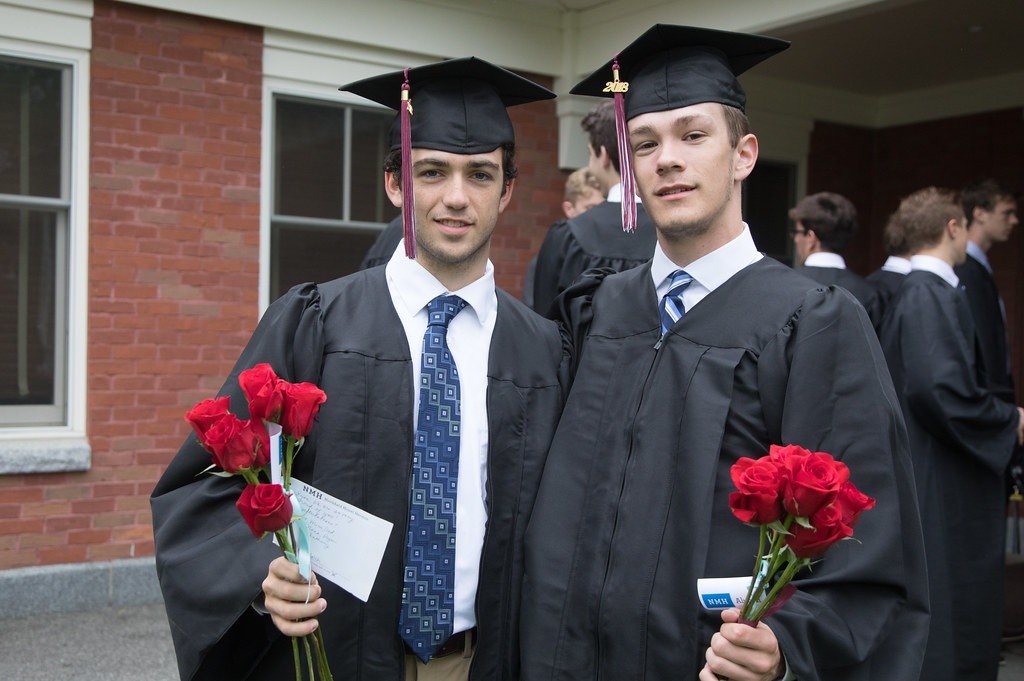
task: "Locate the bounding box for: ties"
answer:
[399,293,468,664]
[658,270,693,336]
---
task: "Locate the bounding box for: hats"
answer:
[339,55,559,260]
[568,23,793,233]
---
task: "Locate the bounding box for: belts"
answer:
[432,625,477,658]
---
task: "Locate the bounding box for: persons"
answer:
[147,85,564,681]
[523,96,657,378]
[788,174,1024,681]
[502,55,902,680]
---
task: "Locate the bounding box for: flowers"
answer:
[726,443,878,628]
[183,360,335,681]
